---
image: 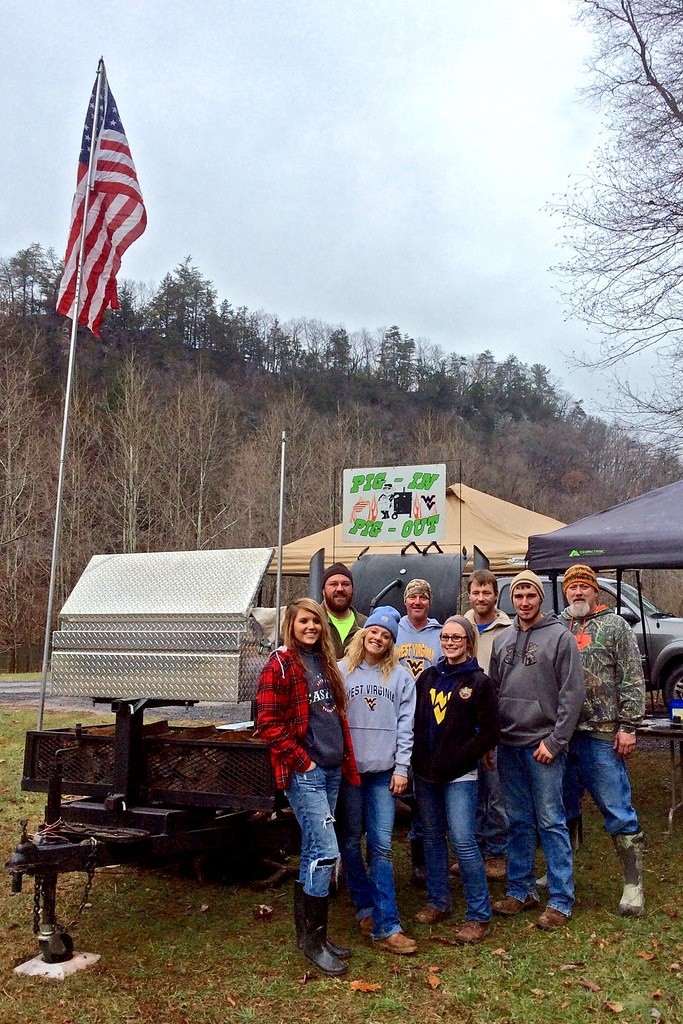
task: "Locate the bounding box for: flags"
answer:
[55,58,148,338]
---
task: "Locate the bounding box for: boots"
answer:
[302,885,350,975]
[536,811,583,887]
[293,879,350,959]
[611,824,645,915]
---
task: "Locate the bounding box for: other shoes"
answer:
[372,931,418,954]
[360,915,375,937]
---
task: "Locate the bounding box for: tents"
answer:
[257,482,683,614]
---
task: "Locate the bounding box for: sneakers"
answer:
[455,919,492,945]
[412,903,455,924]
[536,907,569,932]
[492,894,539,916]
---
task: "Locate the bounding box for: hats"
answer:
[562,564,600,596]
[322,562,354,589]
[509,569,545,605]
[364,605,401,643]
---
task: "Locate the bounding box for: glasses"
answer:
[440,634,469,642]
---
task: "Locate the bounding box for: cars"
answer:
[488,576,682,710]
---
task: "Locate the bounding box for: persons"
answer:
[482,570,586,928]
[255,598,362,975]
[556,564,646,917]
[317,563,513,954]
